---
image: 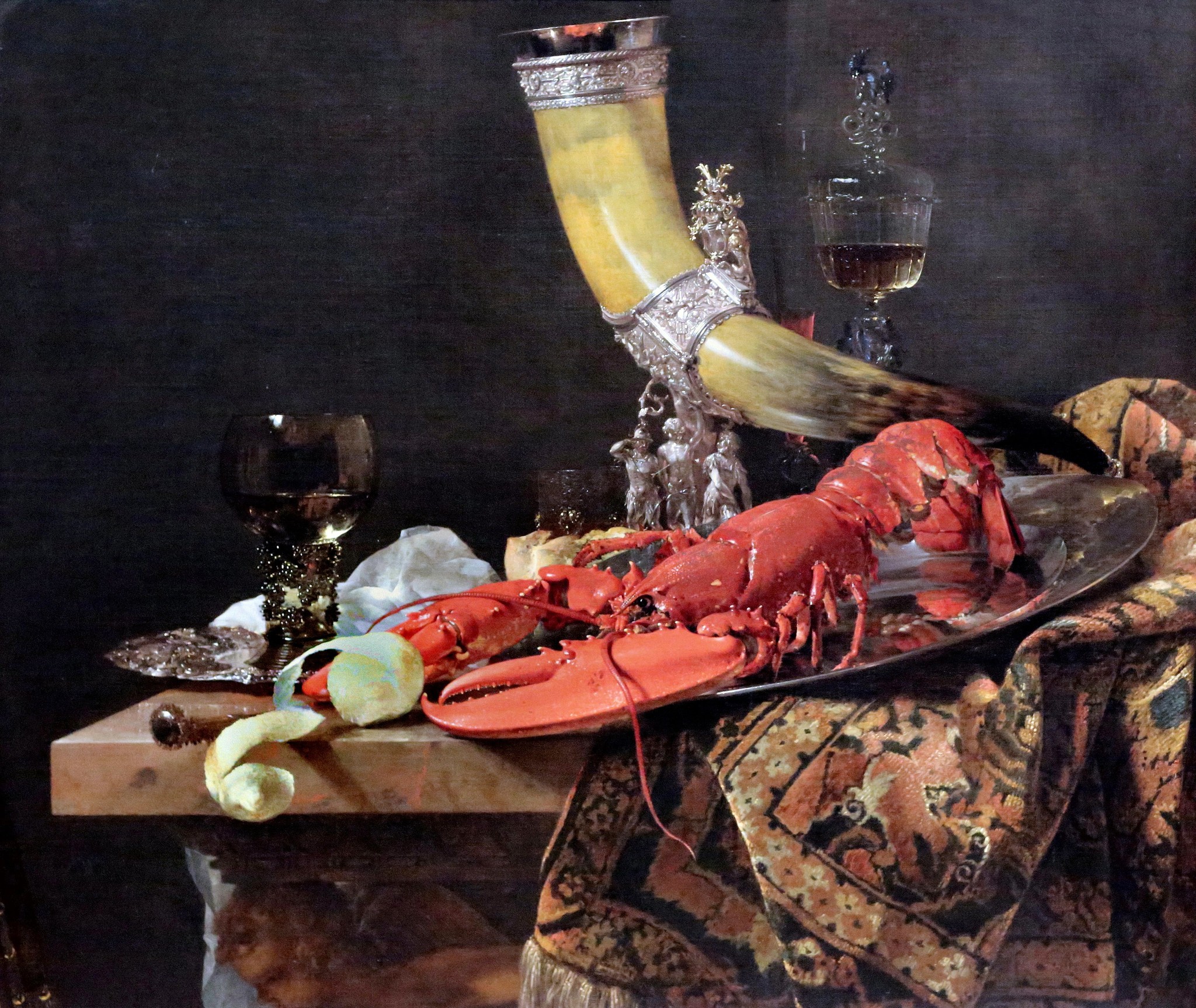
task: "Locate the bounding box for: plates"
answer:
[594,474,1159,697]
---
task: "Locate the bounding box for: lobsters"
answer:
[298,418,1025,860]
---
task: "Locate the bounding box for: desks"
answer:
[47,689,572,1008]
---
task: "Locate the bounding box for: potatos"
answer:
[327,631,425,728]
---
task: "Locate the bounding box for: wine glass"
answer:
[217,412,376,684]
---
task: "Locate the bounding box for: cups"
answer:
[536,466,629,539]
